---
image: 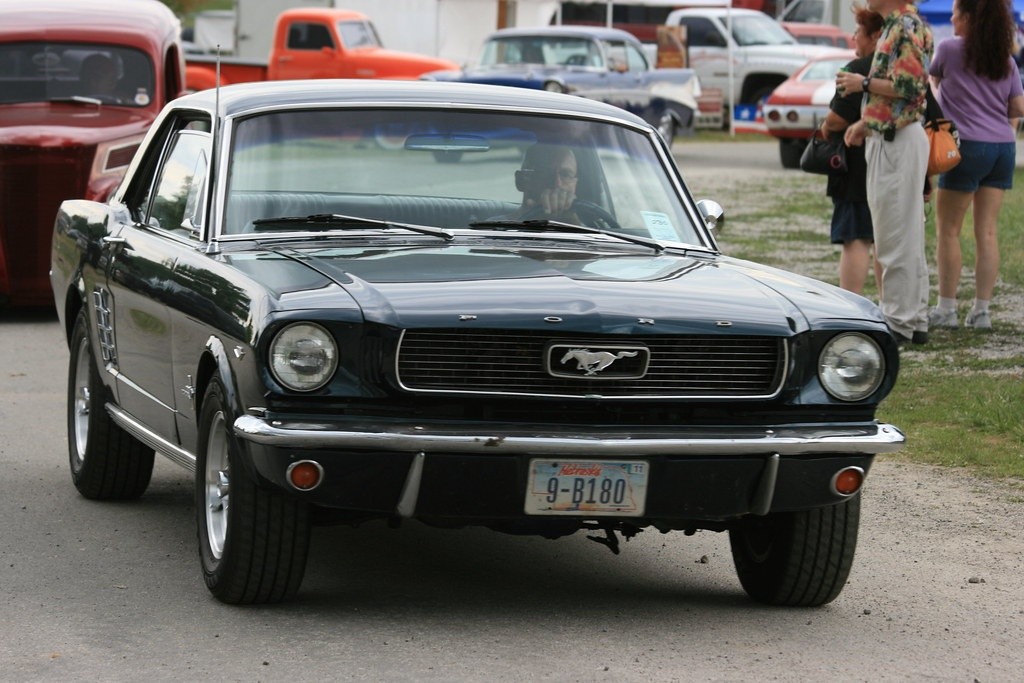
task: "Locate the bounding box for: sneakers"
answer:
[926,305,961,328]
[964,306,993,329]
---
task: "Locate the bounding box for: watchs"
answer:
[862,77,873,94]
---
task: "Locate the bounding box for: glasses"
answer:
[520,168,579,186]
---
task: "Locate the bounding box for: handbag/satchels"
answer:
[800,128,844,174]
[920,117,962,175]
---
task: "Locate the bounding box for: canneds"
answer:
[837,67,851,94]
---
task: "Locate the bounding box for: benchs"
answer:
[194,183,524,234]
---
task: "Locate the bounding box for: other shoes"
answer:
[892,328,928,344]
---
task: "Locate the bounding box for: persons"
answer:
[820,0,1024,346]
[586,41,628,73]
[481,141,588,232]
[74,54,133,104]
